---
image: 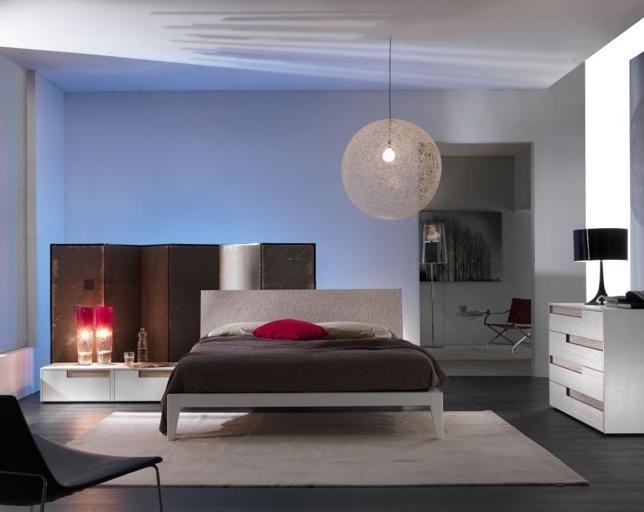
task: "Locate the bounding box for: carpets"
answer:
[64,410,588,483]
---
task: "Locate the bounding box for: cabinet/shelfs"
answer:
[39,364,175,403]
[550,304,643,434]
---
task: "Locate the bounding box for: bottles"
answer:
[136,327,149,363]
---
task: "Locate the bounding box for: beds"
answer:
[158,290,447,440]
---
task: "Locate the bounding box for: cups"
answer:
[123,352,135,366]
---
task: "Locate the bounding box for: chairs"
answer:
[484,300,532,354]
[0,396,163,511]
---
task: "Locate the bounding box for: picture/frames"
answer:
[419,210,503,280]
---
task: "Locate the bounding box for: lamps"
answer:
[97,307,114,364]
[381,37,395,163]
[76,308,94,364]
[574,229,628,305]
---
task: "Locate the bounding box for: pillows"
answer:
[254,320,329,339]
[209,318,394,338]
